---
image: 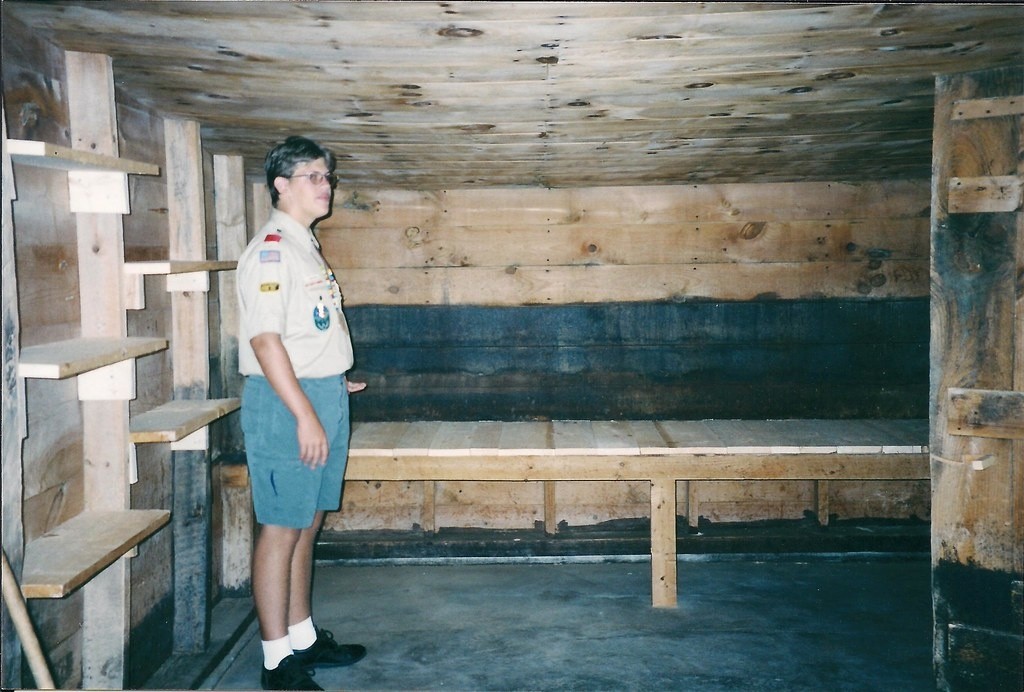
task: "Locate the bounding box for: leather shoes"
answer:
[293,624,367,670]
[260,654,325,691]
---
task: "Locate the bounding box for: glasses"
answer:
[291,171,334,185]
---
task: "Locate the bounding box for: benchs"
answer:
[214,418,931,608]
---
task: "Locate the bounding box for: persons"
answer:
[237,135,367,691]
